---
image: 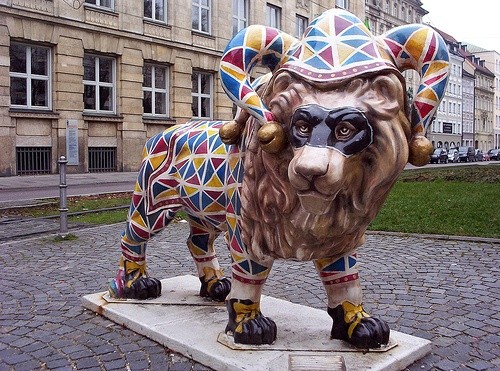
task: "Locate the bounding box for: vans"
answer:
[459,147,476,162]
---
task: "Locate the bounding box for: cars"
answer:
[447,149,460,163]
[487,149,500,161]
[475,149,484,162]
[482,153,490,161]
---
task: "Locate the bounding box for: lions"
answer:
[109,8,451,349]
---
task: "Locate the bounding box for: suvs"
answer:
[431,148,449,163]
